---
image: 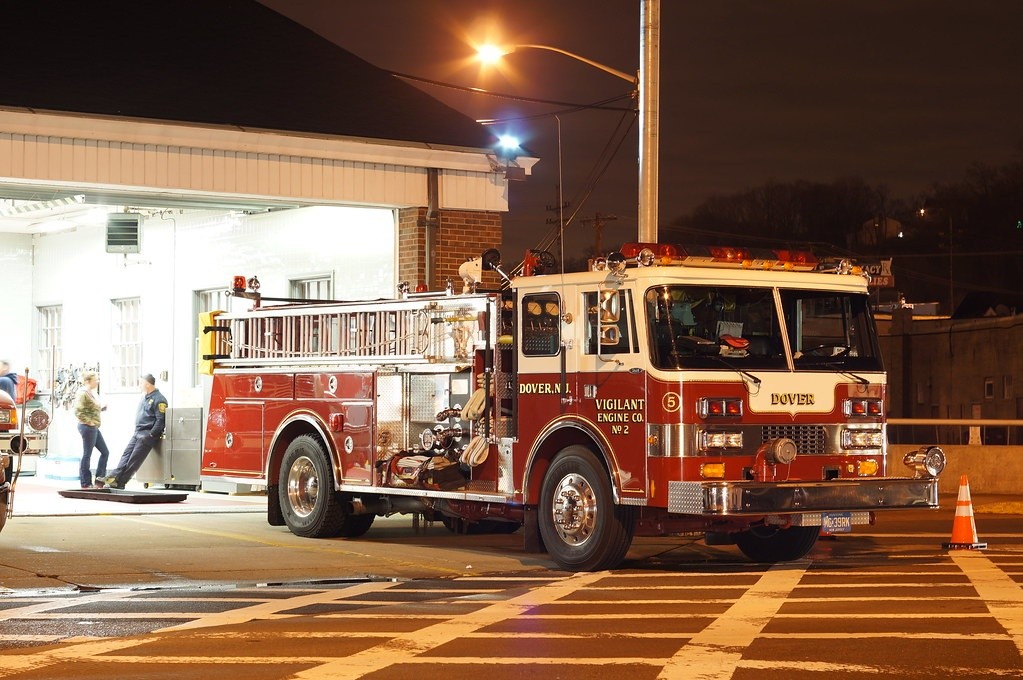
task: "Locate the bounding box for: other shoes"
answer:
[103,478,124,489]
[83,484,93,488]
[97,475,114,484]
[95,482,103,489]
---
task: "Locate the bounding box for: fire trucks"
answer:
[0,388,28,532]
[199,242,946,572]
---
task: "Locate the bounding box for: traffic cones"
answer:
[941,474,987,550]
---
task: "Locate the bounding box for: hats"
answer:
[142,373,155,385]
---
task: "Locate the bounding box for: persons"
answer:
[74,372,109,488]
[96,375,168,490]
[0,359,19,493]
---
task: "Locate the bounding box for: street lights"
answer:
[476,45,658,243]
